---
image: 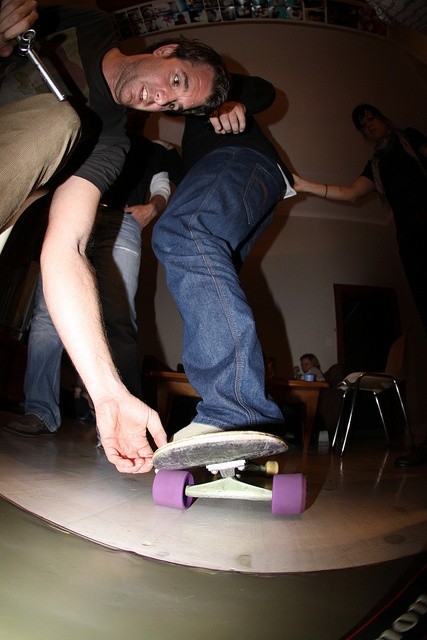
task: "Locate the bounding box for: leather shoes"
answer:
[395,449,427,465]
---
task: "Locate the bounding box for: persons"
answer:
[1,1,228,474]
[292,104,427,467]
[7,138,172,438]
[150,73,294,440]
[300,353,326,381]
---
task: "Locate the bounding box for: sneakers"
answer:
[2,414,57,438]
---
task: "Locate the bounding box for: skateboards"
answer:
[151,430,306,516]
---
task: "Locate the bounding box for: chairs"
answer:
[332,326,415,456]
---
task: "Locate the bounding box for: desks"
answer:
[148,371,329,457]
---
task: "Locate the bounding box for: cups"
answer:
[305,374,315,382]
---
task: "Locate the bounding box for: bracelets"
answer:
[324,184,328,199]
